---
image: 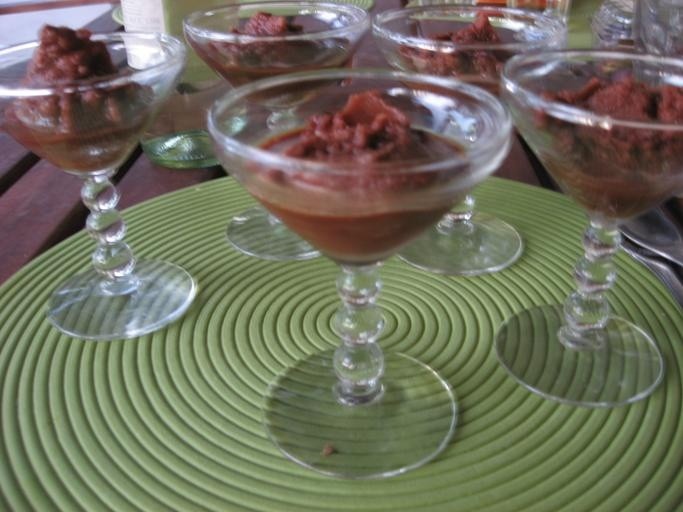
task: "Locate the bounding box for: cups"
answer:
[112,5,236,169]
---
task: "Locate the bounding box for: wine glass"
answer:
[494,49,683,407]
[371,6,568,277]
[182,3,373,262]
[205,68,514,480]
[0,33,196,341]
